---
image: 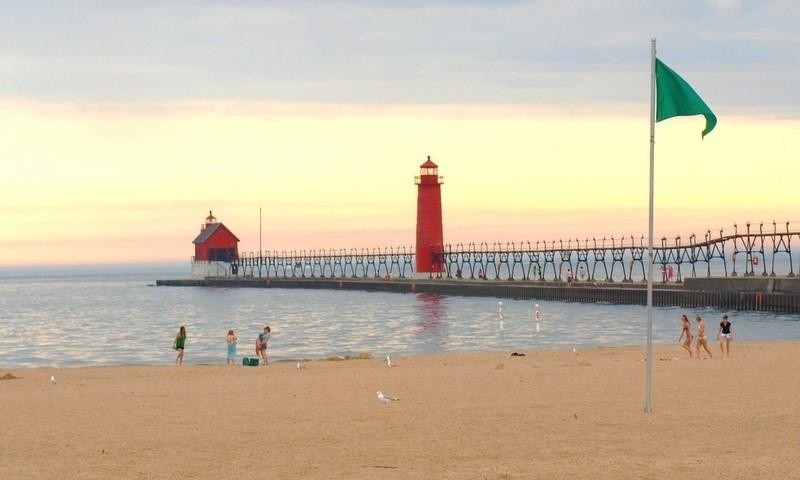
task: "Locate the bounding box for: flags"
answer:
[655,53,718,139]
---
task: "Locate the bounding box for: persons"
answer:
[666,264,675,283]
[579,265,586,281]
[477,269,484,279]
[659,262,669,282]
[715,313,734,357]
[533,265,537,281]
[170,325,188,367]
[693,315,714,360]
[676,313,695,359]
[566,268,574,286]
[224,329,239,365]
[537,264,543,278]
[258,325,272,367]
